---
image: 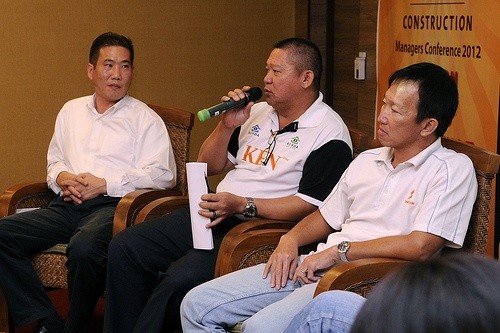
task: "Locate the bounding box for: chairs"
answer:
[1,101,500,328]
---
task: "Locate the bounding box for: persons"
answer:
[103,37,354,333]
[283,248,500,333]
[0,33,178,333]
[180,62,478,333]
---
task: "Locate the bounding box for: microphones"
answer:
[197,87,262,122]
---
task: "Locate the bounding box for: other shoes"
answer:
[64,320,86,333]
[33,317,64,333]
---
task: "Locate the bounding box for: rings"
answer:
[212,211,217,219]
[301,270,305,274]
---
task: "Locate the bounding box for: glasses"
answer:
[262,129,276,166]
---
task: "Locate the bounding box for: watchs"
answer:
[243,197,257,218]
[338,241,351,262]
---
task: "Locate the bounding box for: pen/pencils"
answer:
[204,172,215,212]
